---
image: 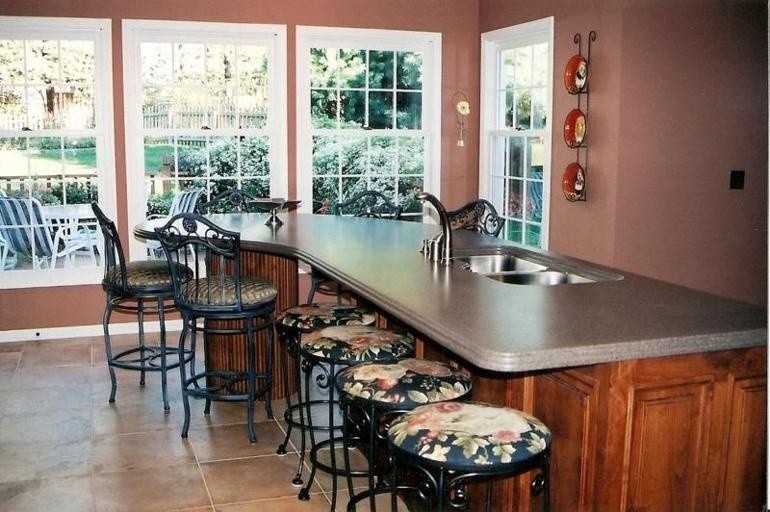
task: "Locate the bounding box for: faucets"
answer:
[415,192,456,267]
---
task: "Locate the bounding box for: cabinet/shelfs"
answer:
[356,290,768,510]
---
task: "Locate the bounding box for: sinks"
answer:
[454,255,548,274]
[485,271,599,287]
